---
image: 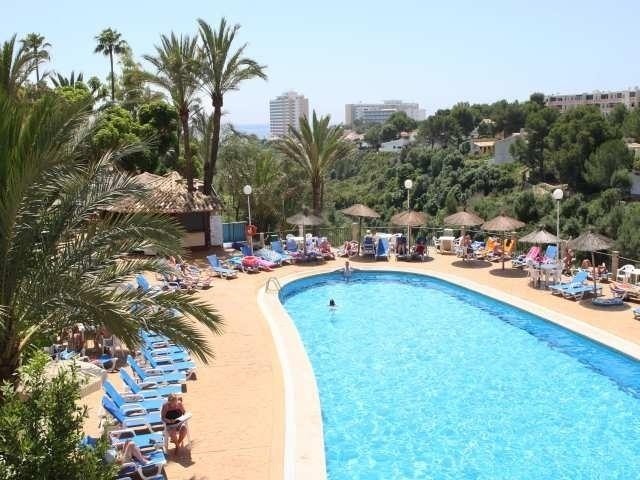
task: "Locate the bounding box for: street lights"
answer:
[403,178,415,255]
[549,188,563,262]
[243,184,255,257]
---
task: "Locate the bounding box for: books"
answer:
[174,412,193,422]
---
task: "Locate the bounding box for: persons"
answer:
[101,434,152,469]
[162,254,194,293]
[320,231,608,279]
[442,210,485,248]
[390,208,428,254]
[161,393,189,459]
[325,298,338,324]
[334,261,362,285]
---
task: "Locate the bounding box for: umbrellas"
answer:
[518,225,565,288]
[567,229,618,299]
[480,215,526,271]
[286,207,325,256]
[339,203,380,255]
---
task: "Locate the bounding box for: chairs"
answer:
[63,259,209,480]
[204,235,457,279]
[456,235,640,321]
